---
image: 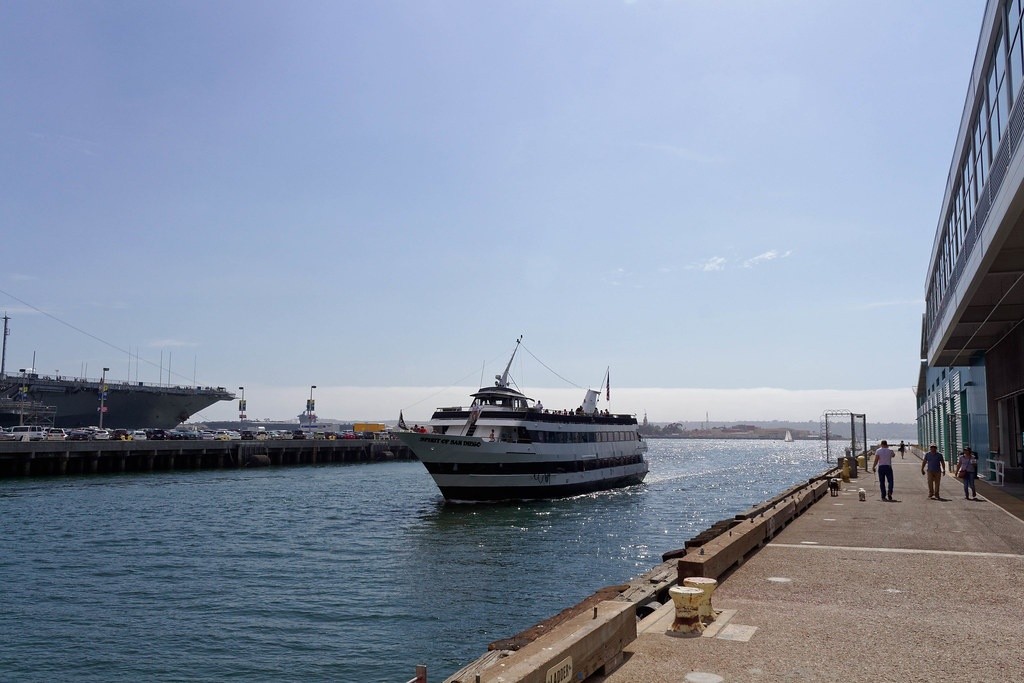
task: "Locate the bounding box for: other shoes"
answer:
[965,497,969,499]
[926,496,933,499]
[887,495,892,500]
[882,498,887,501]
[935,497,940,500]
[972,497,977,500]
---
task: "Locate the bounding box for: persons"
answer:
[413,425,420,433]
[872,440,895,501]
[600,410,603,416]
[418,426,427,433]
[537,400,543,408]
[900,441,905,459]
[592,407,599,416]
[576,406,587,416]
[569,409,574,416]
[489,429,495,442]
[955,447,976,499]
[564,409,567,415]
[512,428,518,443]
[921,443,945,500]
[604,409,609,417]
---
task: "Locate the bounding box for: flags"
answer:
[399,413,409,430]
[607,372,609,401]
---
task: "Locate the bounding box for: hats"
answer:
[964,447,971,454]
[930,443,937,447]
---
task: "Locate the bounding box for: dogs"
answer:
[828,478,839,497]
[857,488,866,501]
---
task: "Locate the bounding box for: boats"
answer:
[0,310,240,430]
[391,334,651,509]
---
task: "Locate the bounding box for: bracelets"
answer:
[943,470,945,471]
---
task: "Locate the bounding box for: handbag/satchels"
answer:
[898,447,901,451]
[959,470,967,478]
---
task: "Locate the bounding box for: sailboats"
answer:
[785,428,794,443]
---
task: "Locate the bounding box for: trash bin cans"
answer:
[847,457,858,478]
[959,451,980,479]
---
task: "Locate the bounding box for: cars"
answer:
[48,428,67,441]
[65,426,392,441]
[0,424,52,442]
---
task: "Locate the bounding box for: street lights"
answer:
[239,387,244,433]
[19,368,26,425]
[309,385,317,432]
[99,367,110,429]
